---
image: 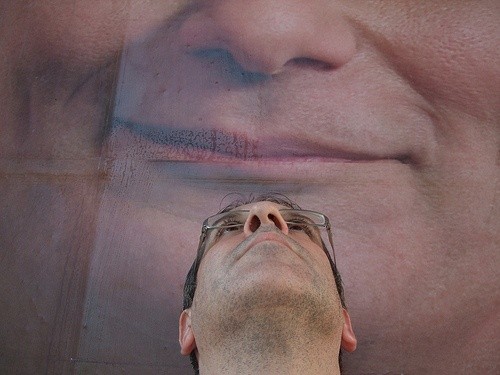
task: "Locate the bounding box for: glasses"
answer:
[193,208,337,277]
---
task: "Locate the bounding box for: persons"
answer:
[0,0,500,375]
[177,189,358,375]
